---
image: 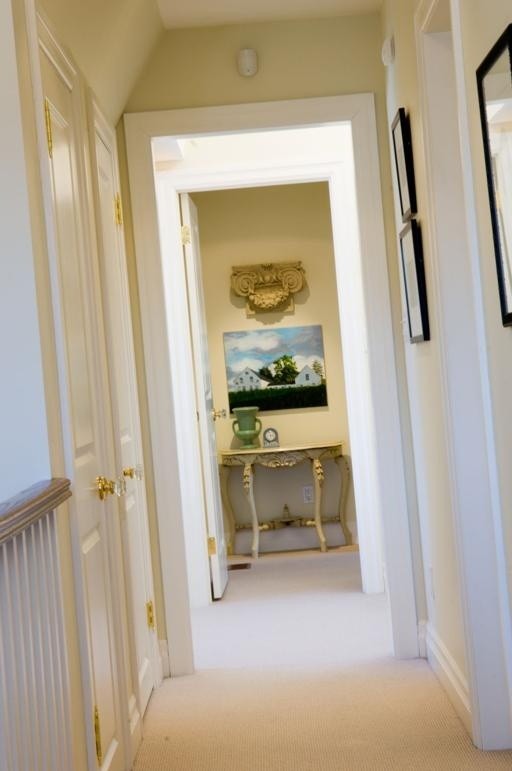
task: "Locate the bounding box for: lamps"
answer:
[235,47,260,76]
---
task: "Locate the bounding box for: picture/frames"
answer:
[474,21,511,329]
[387,104,420,224]
[396,219,431,345]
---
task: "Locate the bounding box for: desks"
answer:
[216,441,352,563]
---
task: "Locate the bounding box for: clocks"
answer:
[260,427,281,450]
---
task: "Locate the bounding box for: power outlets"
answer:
[302,485,314,505]
